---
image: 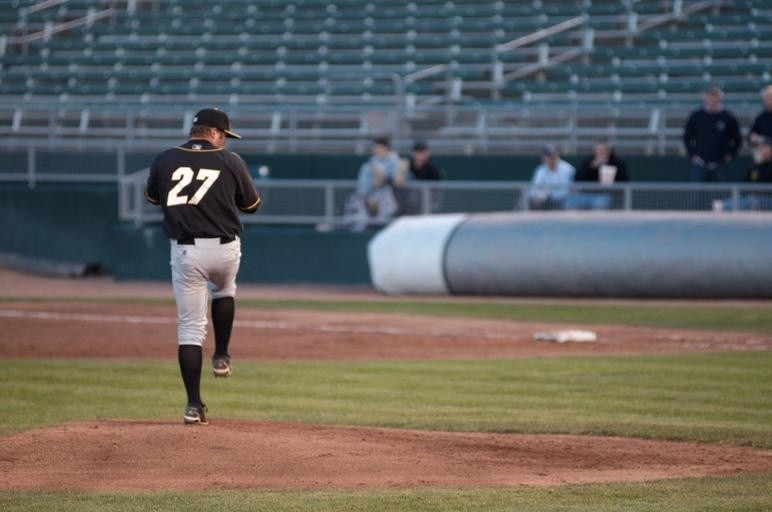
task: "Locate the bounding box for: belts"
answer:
[173,236,238,245]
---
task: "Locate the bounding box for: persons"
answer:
[143,108,263,424]
[356,134,446,214]
[529,133,629,210]
[682,84,772,210]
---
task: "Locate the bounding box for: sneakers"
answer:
[183,402,209,426]
[211,353,232,378]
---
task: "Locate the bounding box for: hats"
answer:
[192,107,242,141]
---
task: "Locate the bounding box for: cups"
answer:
[598,164,618,184]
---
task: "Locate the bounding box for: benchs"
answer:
[2,1,770,210]
